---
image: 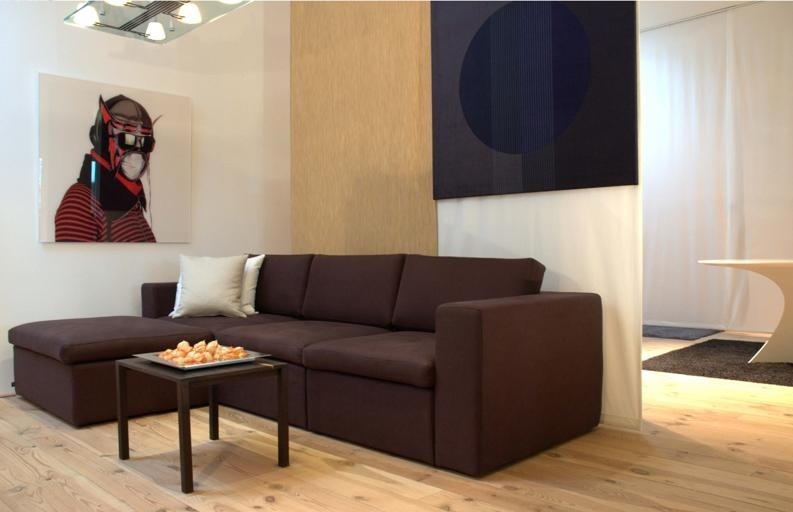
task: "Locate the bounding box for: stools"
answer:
[9,316,214,428]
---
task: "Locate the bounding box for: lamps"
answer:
[63,1,254,44]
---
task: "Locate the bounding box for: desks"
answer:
[698,259,793,364]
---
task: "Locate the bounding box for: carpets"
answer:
[642,339,793,388]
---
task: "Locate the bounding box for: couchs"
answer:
[141,281,603,479]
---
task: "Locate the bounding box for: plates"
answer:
[131,344,272,372]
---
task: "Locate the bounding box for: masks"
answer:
[119,155,145,181]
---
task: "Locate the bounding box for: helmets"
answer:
[92,92,158,165]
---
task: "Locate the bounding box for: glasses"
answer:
[113,133,157,153]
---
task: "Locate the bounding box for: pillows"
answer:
[171,254,266,319]
[170,253,247,318]
